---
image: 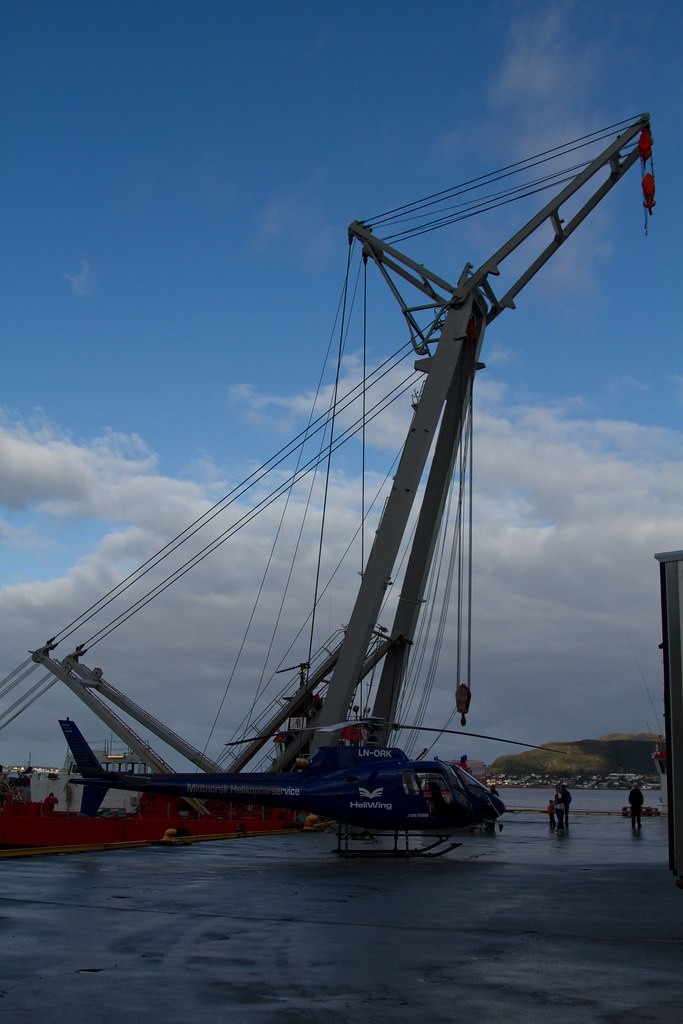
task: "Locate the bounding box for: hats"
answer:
[560,782,567,787]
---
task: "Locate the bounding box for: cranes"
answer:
[0,113,656,857]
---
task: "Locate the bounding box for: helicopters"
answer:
[57,717,568,857]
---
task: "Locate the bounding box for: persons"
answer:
[485,785,499,831]
[547,785,572,832]
[629,785,643,828]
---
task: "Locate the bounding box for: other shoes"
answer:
[638,824,641,828]
[554,825,556,829]
[632,824,635,828]
[550,830,554,833]
[560,825,564,829]
[557,826,561,829]
[566,825,569,828]
